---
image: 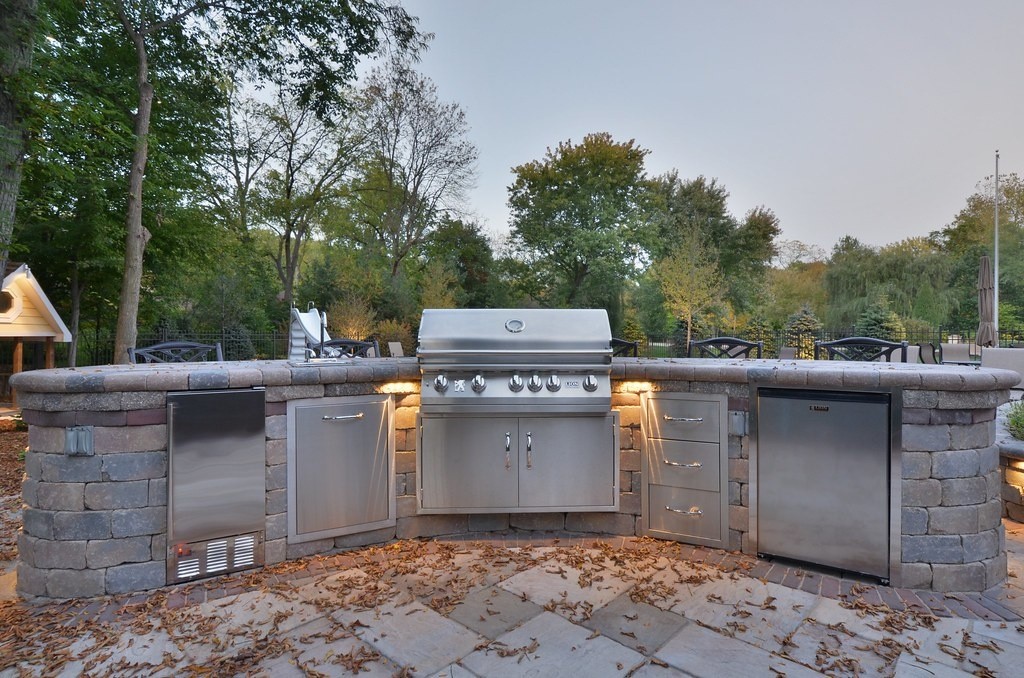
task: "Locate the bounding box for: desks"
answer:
[943,360,981,366]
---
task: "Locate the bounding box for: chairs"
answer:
[127,341,223,364]
[687,338,762,358]
[916,342,938,364]
[815,337,908,363]
[940,343,971,361]
[611,338,638,358]
